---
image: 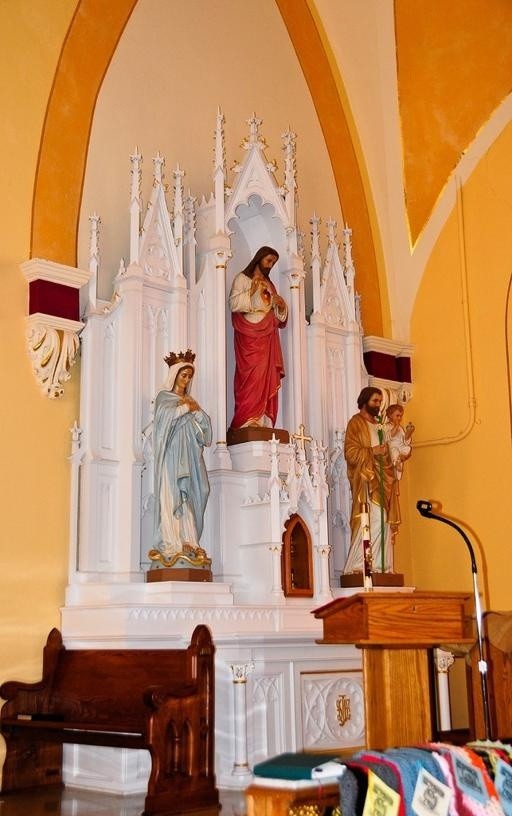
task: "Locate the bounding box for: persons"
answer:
[345,386,389,575]
[229,246,287,426]
[383,403,414,477]
[152,352,212,567]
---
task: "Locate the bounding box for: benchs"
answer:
[0,624,218,816]
